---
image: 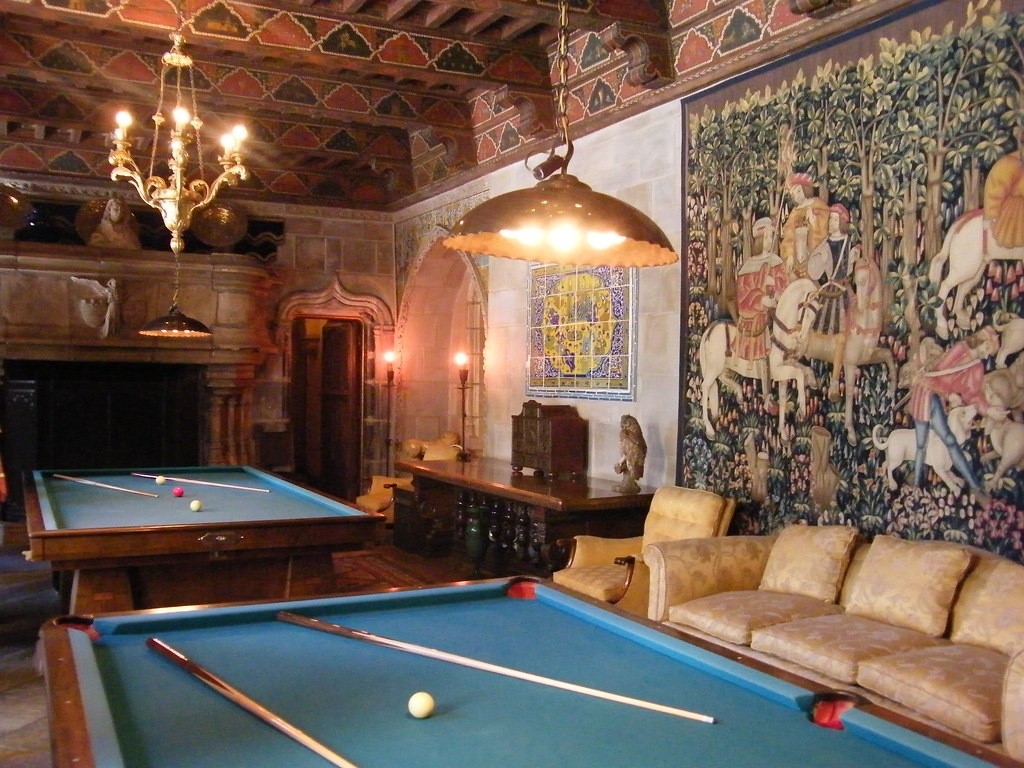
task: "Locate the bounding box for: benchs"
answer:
[642,535,1024,768]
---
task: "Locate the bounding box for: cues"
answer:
[277,610,714,724]
[131,472,270,492]
[147,637,357,768]
[53,474,159,498]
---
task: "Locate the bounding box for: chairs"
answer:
[552,484,736,603]
[356,430,463,524]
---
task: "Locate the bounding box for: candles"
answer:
[453,351,469,382]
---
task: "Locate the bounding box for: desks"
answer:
[389,454,659,573]
[39,570,1020,768]
[21,462,387,619]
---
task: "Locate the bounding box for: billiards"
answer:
[408,692,434,719]
[156,476,165,484]
[190,500,202,512]
[173,486,184,497]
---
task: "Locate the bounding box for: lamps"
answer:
[107,1,251,339]
[442,0,681,268]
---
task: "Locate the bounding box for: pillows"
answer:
[755,525,859,604]
[844,534,973,637]
